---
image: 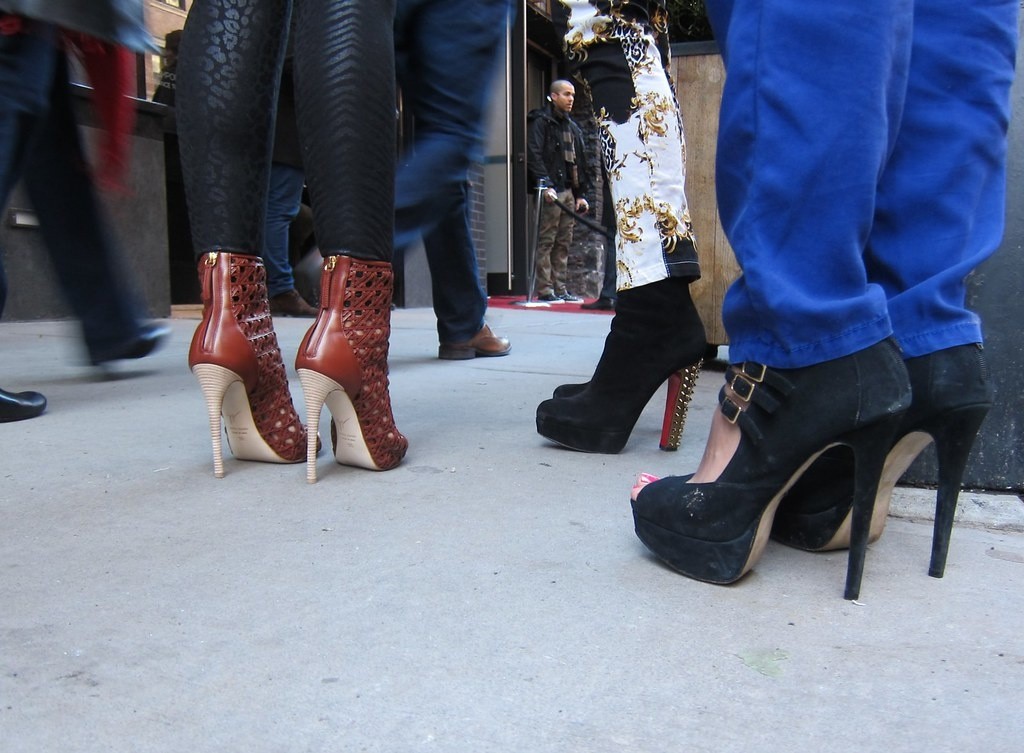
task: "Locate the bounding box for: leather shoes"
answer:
[439,322,512,359]
[581,297,611,311]
[269,289,321,320]
[89,323,168,367]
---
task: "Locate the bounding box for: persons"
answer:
[531,2,704,463]
[389,0,519,362]
[532,78,592,305]
[612,1,1024,604]
[263,37,321,319]
[2,0,180,429]
[172,1,411,486]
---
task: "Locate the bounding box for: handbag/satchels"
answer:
[9,0,162,57]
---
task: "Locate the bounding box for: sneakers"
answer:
[556,292,584,304]
[538,294,566,305]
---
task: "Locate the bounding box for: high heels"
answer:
[769,341,990,585]
[187,253,322,478]
[294,256,409,484]
[536,279,707,457]
[630,337,911,602]
[0,387,46,423]
[554,381,591,401]
